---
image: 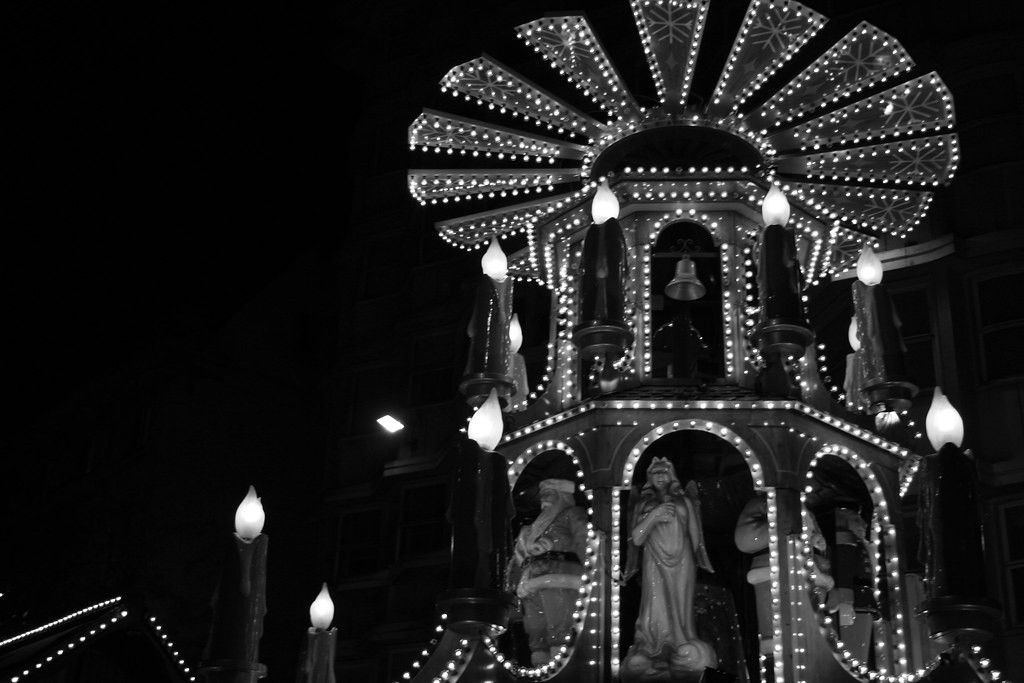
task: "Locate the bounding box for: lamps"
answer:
[762,182,790,226]
[234,485,266,538]
[481,235,508,282]
[310,582,334,631]
[509,312,523,354]
[857,243,883,286]
[592,178,620,225]
[468,386,504,451]
[925,386,964,451]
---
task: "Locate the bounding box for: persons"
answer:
[503,479,596,671]
[619,456,718,683]
[733,482,854,683]
[685,479,704,533]
